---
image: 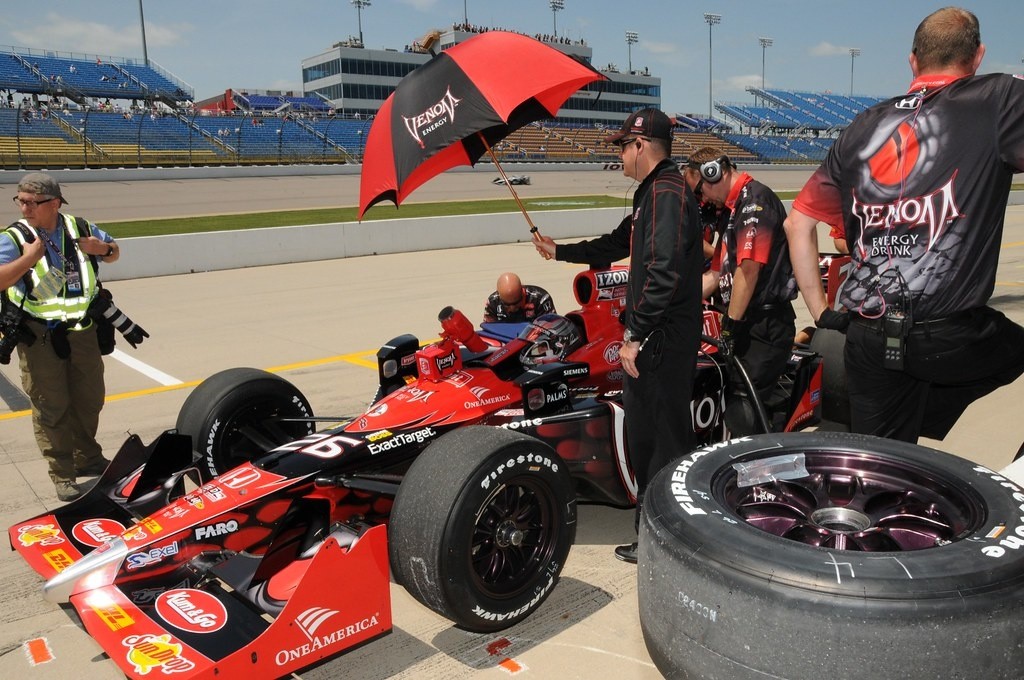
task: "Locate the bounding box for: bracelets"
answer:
[100,245,113,257]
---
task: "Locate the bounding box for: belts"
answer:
[852,305,987,336]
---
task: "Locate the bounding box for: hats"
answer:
[17,172,68,206]
[603,107,674,144]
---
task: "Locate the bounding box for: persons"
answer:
[99,97,235,120]
[0,171,120,502]
[782,4,1024,445]
[413,41,421,51]
[807,97,824,106]
[0,93,73,123]
[682,147,798,442]
[348,35,357,44]
[454,22,584,45]
[70,59,128,88]
[698,199,726,311]
[519,314,582,371]
[243,109,268,125]
[218,127,232,135]
[297,113,318,122]
[328,108,361,120]
[484,272,557,322]
[33,63,62,82]
[531,108,704,564]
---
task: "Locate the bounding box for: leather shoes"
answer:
[615,542,638,564]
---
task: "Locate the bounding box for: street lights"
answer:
[703,12,722,119]
[626,30,640,73]
[848,48,861,96]
[760,37,773,89]
[350,0,373,44]
[547,0,565,38]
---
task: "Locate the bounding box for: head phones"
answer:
[699,155,731,184]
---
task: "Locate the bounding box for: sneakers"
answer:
[52,476,81,501]
[74,456,111,475]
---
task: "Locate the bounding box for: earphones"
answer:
[636,140,641,149]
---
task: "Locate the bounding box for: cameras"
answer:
[87,289,149,348]
[0,311,36,364]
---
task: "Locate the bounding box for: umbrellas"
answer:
[358,30,611,260]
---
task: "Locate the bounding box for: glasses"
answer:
[12,195,53,210]
[500,291,525,307]
[693,178,706,203]
[618,135,653,153]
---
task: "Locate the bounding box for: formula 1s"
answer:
[5,257,825,680]
[492,175,531,187]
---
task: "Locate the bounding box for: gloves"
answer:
[716,313,747,365]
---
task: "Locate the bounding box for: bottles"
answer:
[47,320,71,358]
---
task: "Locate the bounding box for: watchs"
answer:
[623,329,640,343]
